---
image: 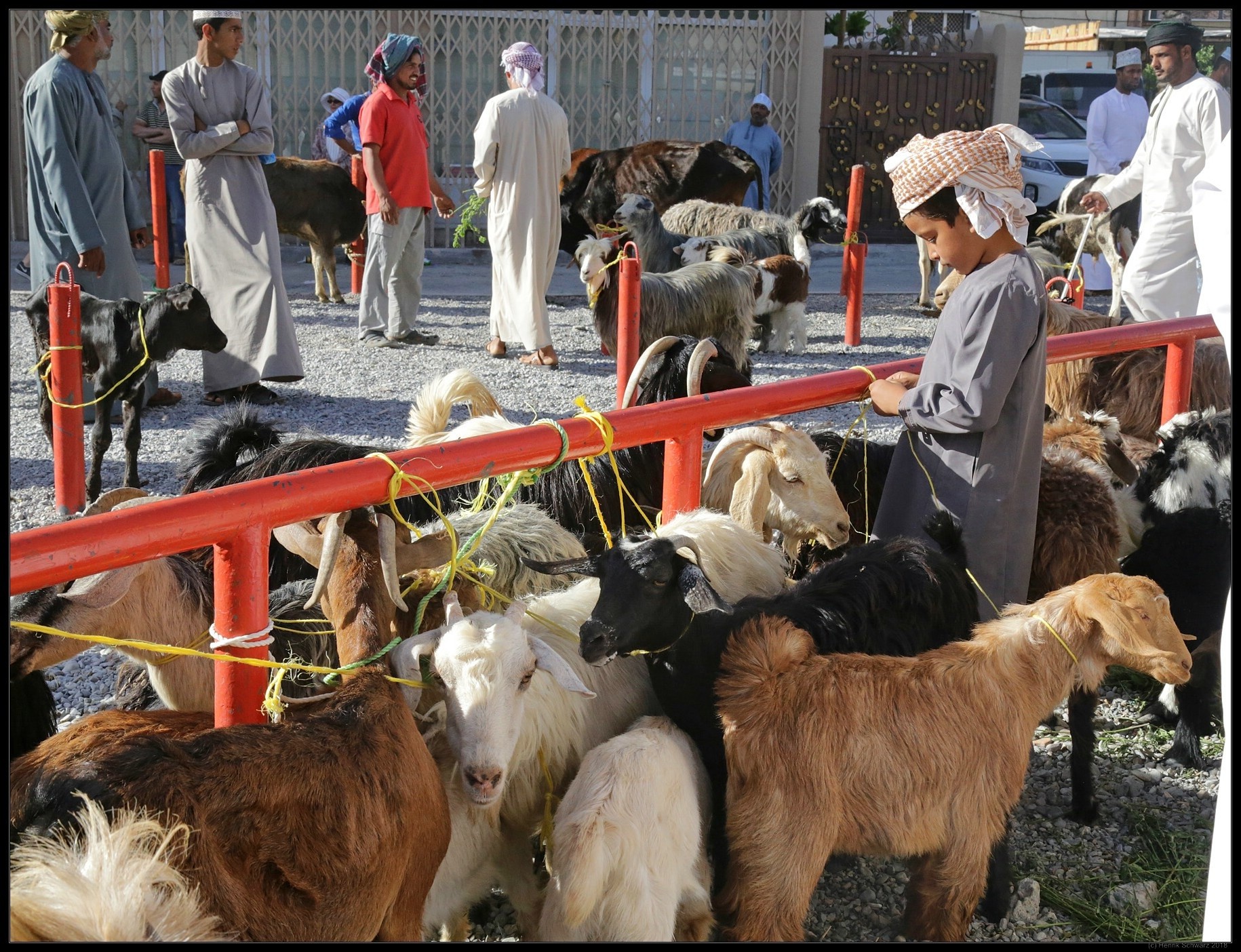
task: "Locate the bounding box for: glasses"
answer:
[327,99,341,103]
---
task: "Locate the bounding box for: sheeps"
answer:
[612,187,849,355]
[7,335,1233,952]
[713,566,1195,943]
[932,264,1231,442]
[567,232,758,367]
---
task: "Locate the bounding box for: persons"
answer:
[159,0,306,408]
[1078,20,1241,360]
[12,0,186,421]
[868,117,1047,924]
[311,31,455,349]
[471,42,572,370]
[725,93,784,212]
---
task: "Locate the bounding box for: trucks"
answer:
[1018,49,1147,135]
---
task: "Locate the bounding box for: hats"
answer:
[149,70,168,80]
[1145,20,1205,49]
[320,88,350,114]
[752,93,772,111]
[1221,47,1230,62]
[1115,47,1141,69]
[192,10,242,21]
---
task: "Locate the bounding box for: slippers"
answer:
[394,331,439,344]
[486,343,506,357]
[518,351,554,368]
[239,384,287,404]
[360,336,397,348]
[202,391,235,404]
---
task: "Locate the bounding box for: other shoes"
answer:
[84,416,124,424]
[174,258,186,265]
[146,388,181,405]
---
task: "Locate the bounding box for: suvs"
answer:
[1016,92,1088,238]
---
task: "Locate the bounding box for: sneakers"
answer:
[14,262,31,280]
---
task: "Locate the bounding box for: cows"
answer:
[917,172,1141,318]
[179,157,369,304]
[24,276,228,507]
[559,138,764,256]
